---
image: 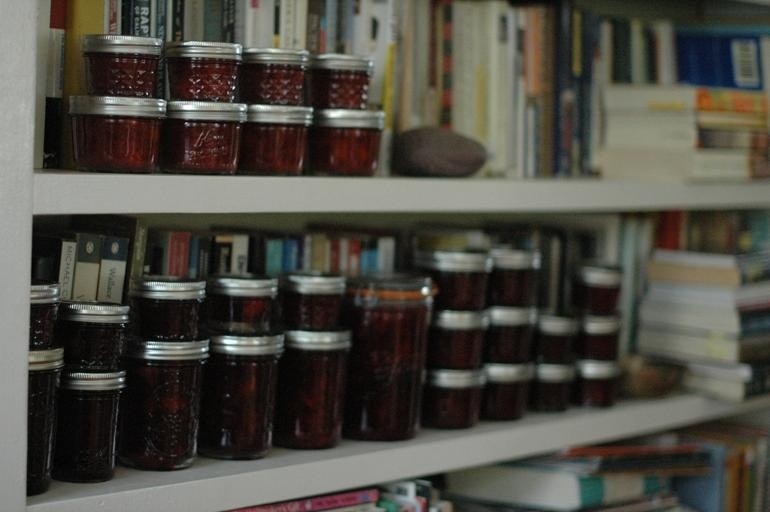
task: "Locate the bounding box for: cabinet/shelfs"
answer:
[0,0,770,511]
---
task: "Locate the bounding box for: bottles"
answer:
[305,51,377,110]
[418,250,622,431]
[126,273,207,343]
[51,370,127,484]
[275,332,352,451]
[210,276,281,339]
[80,33,161,96]
[347,274,438,443]
[238,46,309,105]
[117,342,195,472]
[241,104,315,175]
[27,348,67,495]
[163,100,246,173]
[309,106,385,175]
[164,40,244,102]
[29,284,61,351]
[70,97,167,175]
[196,336,282,462]
[284,271,352,332]
[55,299,131,373]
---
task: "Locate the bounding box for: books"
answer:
[34,0,770,512]
